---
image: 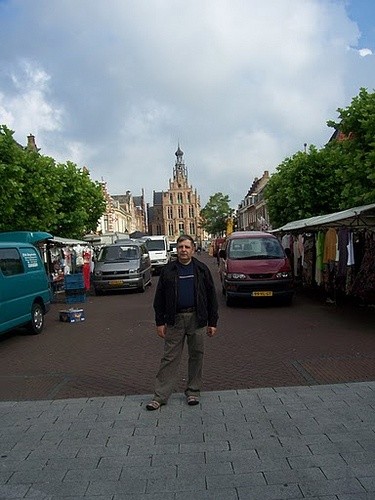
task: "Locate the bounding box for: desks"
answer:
[48,279,63,303]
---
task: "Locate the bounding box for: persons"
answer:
[147,234,219,410]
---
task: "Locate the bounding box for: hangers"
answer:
[282,225,368,234]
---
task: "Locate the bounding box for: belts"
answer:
[176,305,196,313]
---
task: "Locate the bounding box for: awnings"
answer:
[267,204,375,233]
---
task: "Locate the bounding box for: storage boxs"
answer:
[59,311,86,323]
[64,273,86,304]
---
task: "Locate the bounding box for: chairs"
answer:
[243,244,256,256]
[231,244,243,256]
[126,248,137,257]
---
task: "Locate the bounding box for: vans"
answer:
[142,236,173,267]
[217,232,295,306]
[0,231,55,336]
[92,238,152,295]
[168,242,178,256]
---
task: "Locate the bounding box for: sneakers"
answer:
[186,396,199,405]
[146,400,161,410]
